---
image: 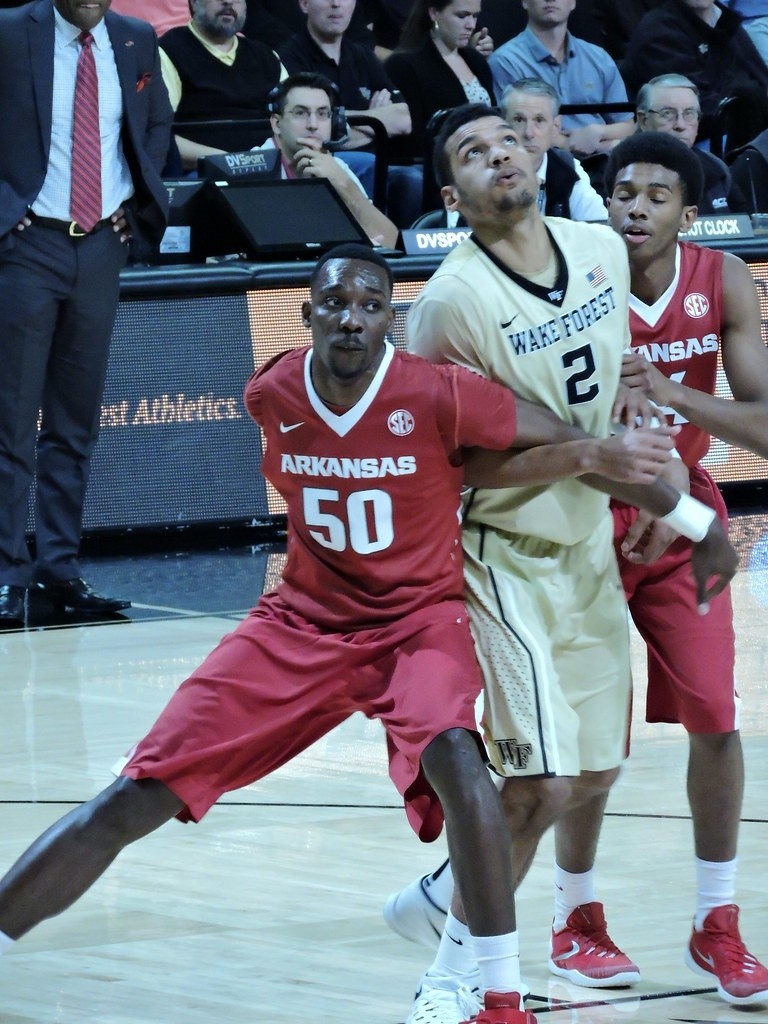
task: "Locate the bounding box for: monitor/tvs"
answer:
[209,175,374,264]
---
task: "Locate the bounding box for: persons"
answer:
[382,105,691,1024]
[0,0,174,626]
[106,0,768,266]
[0,245,741,1024]
[549,135,768,1010]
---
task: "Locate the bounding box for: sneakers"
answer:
[404,971,484,1024]
[457,991,538,1024]
[684,904,768,1005]
[548,902,641,988]
[383,872,531,1002]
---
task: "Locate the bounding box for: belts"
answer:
[25,209,113,237]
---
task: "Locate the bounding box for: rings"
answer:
[308,158,312,166]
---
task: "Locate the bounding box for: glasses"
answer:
[276,107,332,121]
[647,107,702,124]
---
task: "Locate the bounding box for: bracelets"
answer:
[661,494,719,542]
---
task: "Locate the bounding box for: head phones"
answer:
[267,71,347,139]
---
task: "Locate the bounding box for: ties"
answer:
[69,32,103,234]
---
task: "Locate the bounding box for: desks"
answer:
[27,231,768,534]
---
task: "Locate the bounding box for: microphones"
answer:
[323,124,350,150]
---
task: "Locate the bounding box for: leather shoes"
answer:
[0,585,26,628]
[27,578,131,612]
